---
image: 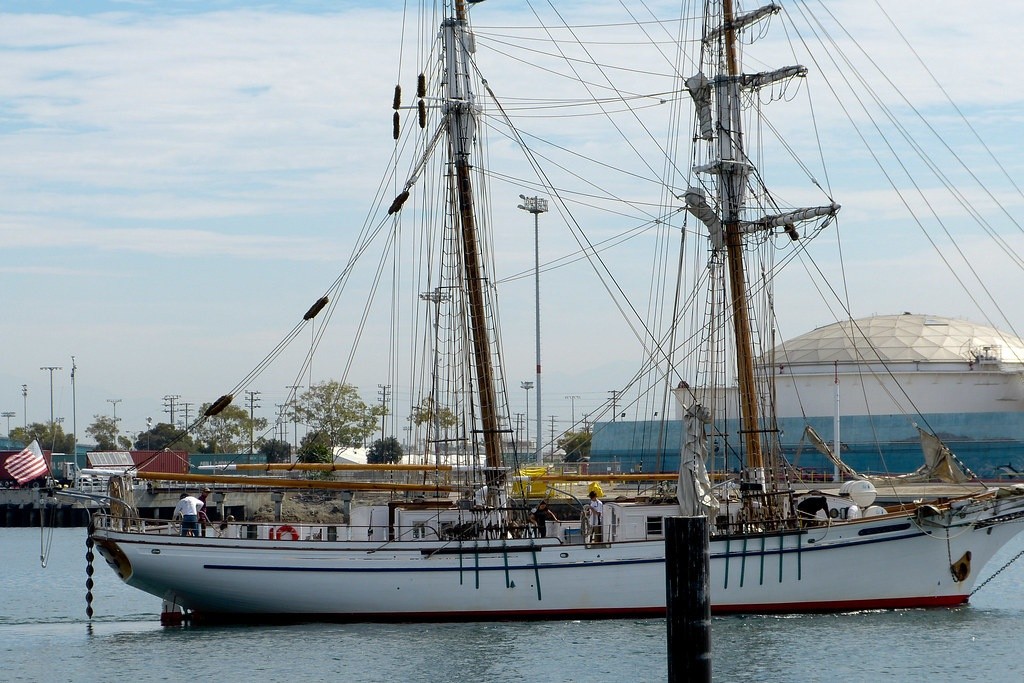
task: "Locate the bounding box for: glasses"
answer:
[205,491,210,494]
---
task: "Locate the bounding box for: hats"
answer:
[203,488,211,492]
[589,491,596,497]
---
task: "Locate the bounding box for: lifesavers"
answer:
[276,526,298,540]
[269,528,279,540]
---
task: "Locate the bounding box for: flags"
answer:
[5,439,47,485]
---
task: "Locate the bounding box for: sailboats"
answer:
[34,0,1024,629]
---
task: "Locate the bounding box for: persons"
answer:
[174,493,204,536]
[587,491,603,542]
[530,501,558,537]
[457,490,471,509]
[198,488,210,536]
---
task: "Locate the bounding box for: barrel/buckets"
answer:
[863,504,888,517]
[840,481,877,508]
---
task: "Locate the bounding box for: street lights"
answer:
[106,399,123,446]
[564,396,582,433]
[418,284,450,472]
[145,416,152,453]
[42,366,63,425]
[520,381,534,462]
[20,384,29,432]
[1,411,16,436]
[126,430,143,445]
[518,190,551,464]
